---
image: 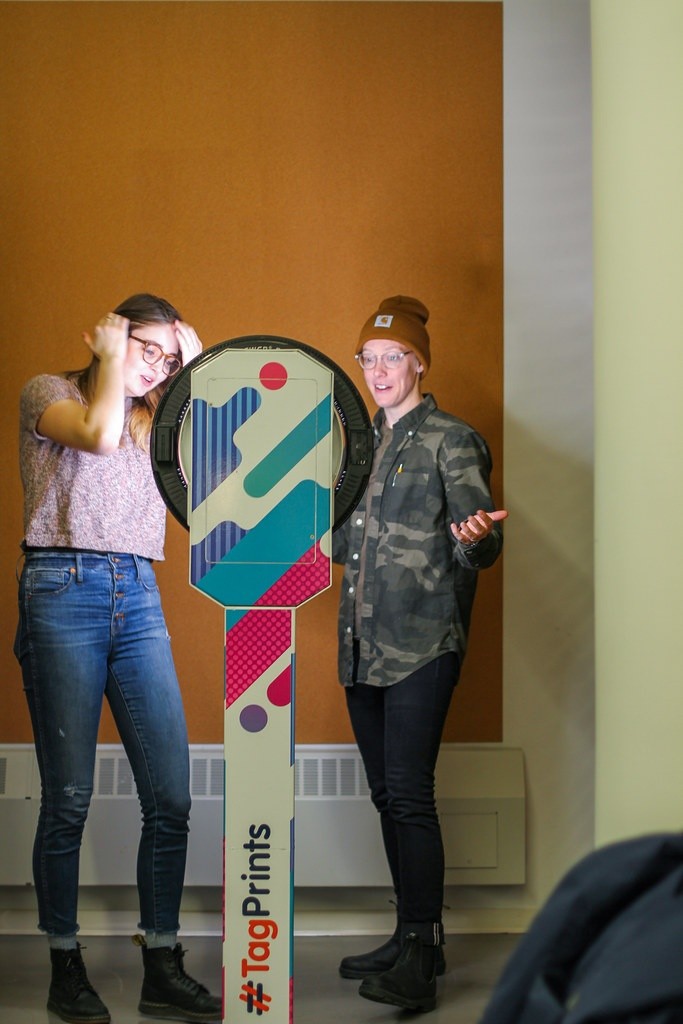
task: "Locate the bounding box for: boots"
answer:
[130,933,223,1021]
[45,941,112,1024]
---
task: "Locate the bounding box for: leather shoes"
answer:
[338,917,447,978]
[358,922,439,1011]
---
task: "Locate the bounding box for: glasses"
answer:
[129,334,182,377]
[354,348,413,370]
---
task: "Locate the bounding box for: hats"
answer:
[355,295,430,381]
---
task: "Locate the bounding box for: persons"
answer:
[16,294,220,1024]
[332,297,510,1014]
